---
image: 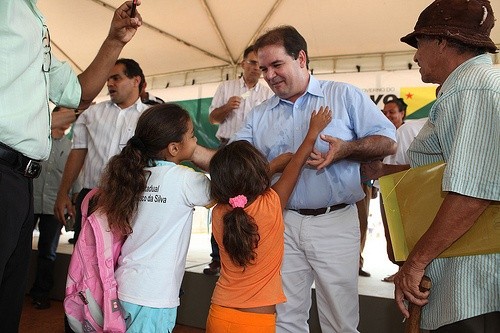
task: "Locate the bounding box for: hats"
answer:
[400,0,497,53]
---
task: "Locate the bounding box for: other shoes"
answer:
[381,272,399,282]
[29,288,49,309]
[359,269,370,276]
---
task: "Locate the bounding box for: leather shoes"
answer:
[204,262,220,275]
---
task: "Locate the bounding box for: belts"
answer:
[0,147,42,179]
[289,203,349,216]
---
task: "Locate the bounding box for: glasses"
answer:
[42,25,51,72]
[242,60,258,65]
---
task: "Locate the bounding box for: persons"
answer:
[355,98,428,281]
[192,26,397,333]
[204,46,276,275]
[0,0,142,333]
[395,1,500,333]
[96,100,294,333]
[206,105,333,333]
[24,57,166,333]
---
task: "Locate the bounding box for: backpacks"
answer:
[63,186,131,333]
